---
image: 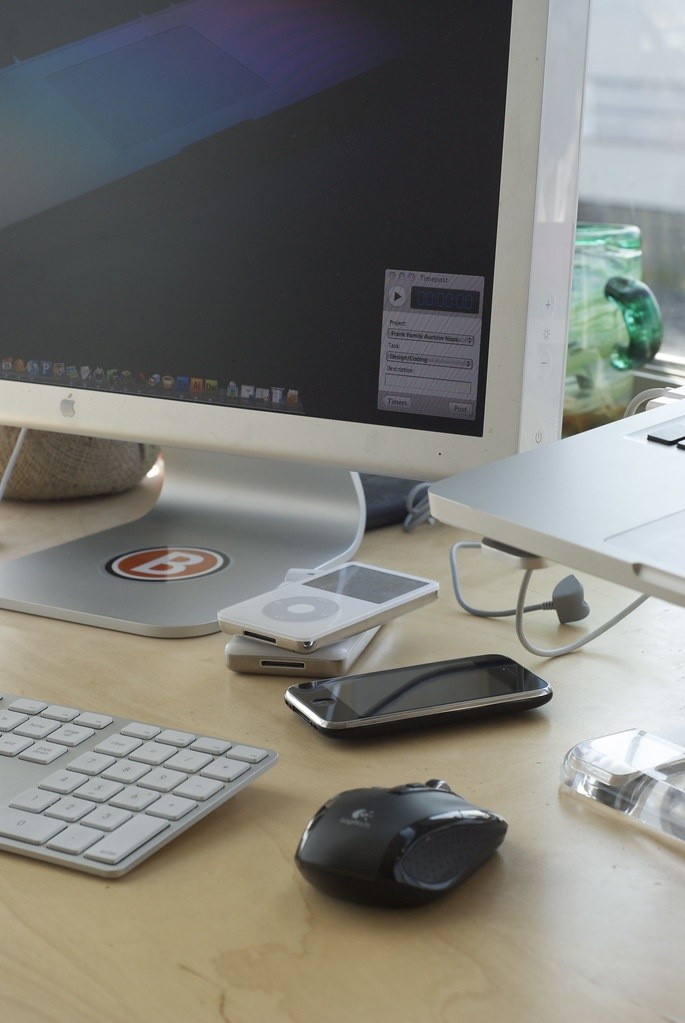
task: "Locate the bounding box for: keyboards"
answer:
[0,693,279,880]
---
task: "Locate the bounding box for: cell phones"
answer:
[283,653,554,741]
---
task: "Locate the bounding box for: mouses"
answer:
[291,777,509,909]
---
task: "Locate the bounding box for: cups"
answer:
[560,225,664,437]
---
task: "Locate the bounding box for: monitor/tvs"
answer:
[3,0,592,650]
